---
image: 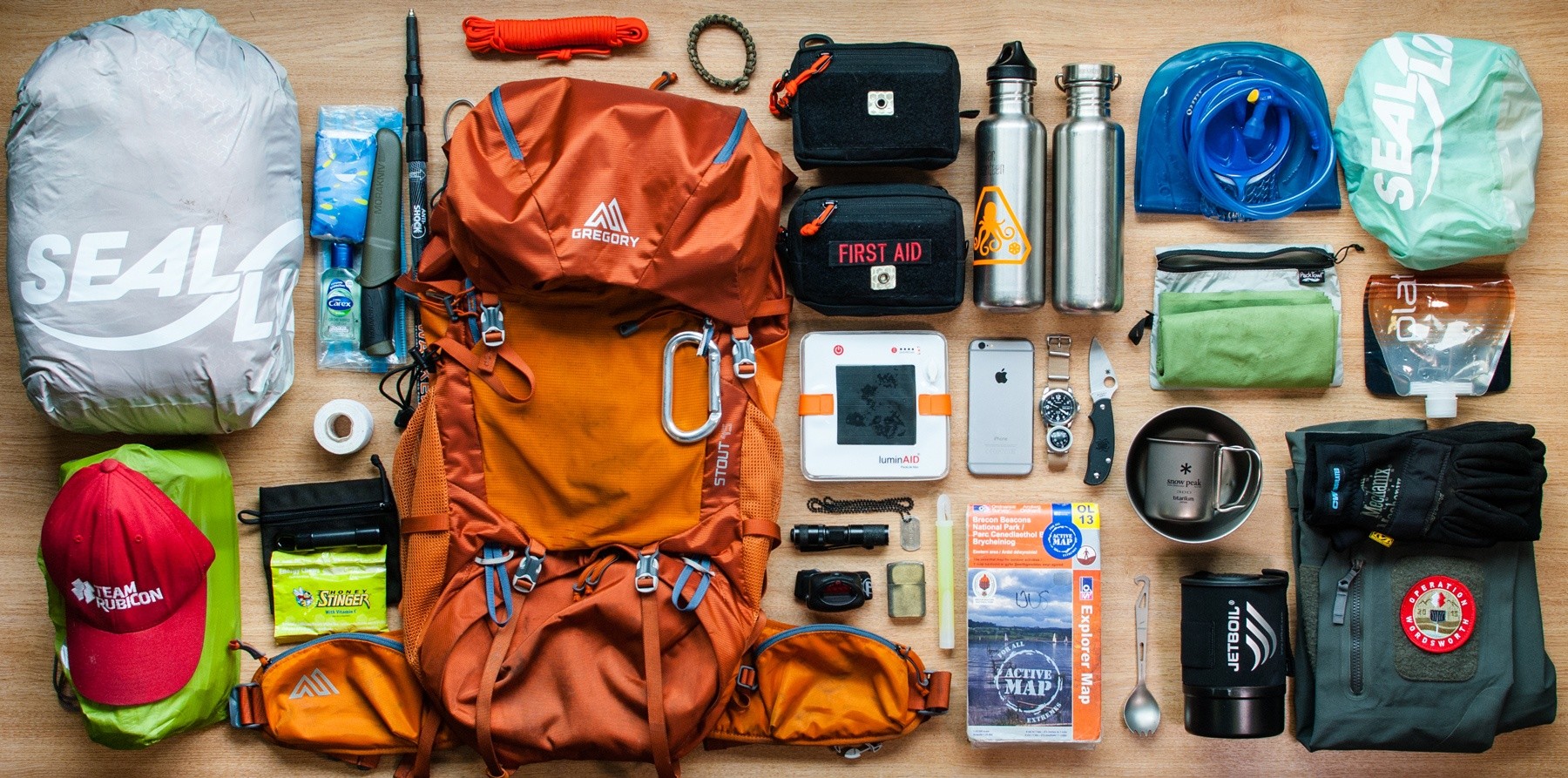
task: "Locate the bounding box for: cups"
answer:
[1146,438,1263,521]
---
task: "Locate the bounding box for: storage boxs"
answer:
[963,500,1104,751]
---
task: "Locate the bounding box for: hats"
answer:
[41,462,216,710]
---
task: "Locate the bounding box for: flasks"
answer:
[1052,62,1127,316]
[975,41,1045,314]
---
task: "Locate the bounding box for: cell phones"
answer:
[968,337,1034,476]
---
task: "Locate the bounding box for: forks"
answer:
[1124,578,1161,737]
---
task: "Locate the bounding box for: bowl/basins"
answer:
[1126,405,1264,543]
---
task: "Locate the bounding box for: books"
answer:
[965,502,1103,753]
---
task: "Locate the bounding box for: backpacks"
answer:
[391,78,794,778]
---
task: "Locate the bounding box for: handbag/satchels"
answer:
[781,35,981,169]
[1147,244,1364,390]
[782,184,969,315]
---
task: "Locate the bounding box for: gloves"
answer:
[1305,421,1547,552]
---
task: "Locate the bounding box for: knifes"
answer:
[1083,338,1117,487]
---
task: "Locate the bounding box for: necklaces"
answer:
[806,496,920,551]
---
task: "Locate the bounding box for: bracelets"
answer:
[686,13,757,92]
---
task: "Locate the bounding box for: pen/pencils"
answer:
[404,6,429,261]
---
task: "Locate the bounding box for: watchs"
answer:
[1039,333,1080,473]
